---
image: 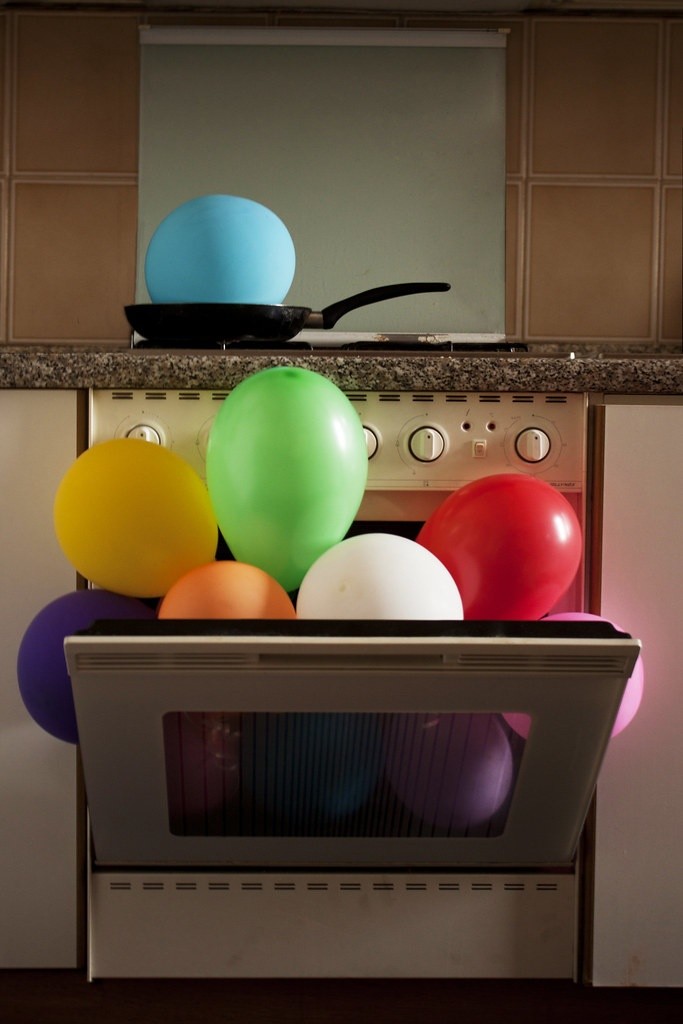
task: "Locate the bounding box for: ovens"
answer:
[78,385,641,986]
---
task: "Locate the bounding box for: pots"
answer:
[123,281,452,341]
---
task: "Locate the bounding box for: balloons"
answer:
[164,711,251,822]
[144,194,297,305]
[502,612,645,741]
[251,712,393,815]
[416,472,582,621]
[205,366,368,593]
[297,533,465,620]
[159,562,299,619]
[16,589,160,747]
[389,713,514,829]
[52,437,219,596]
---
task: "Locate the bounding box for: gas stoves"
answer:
[122,336,534,351]
